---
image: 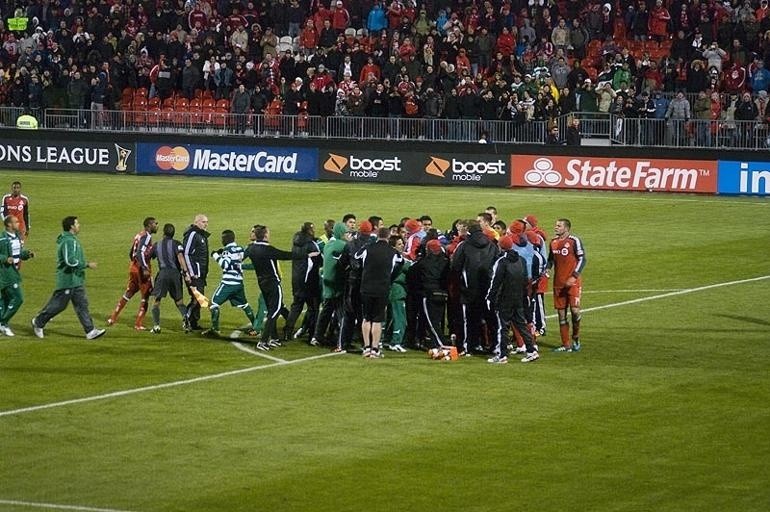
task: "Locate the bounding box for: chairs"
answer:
[111,0,369,136]
[552,1,770,147]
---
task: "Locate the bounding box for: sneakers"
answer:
[293,328,303,339]
[0,325,15,336]
[310,337,319,346]
[108,318,114,324]
[87,328,105,340]
[363,342,406,359]
[150,325,161,333]
[134,324,147,330]
[246,327,291,351]
[572,335,580,351]
[486,343,539,363]
[182,315,220,337]
[458,350,471,357]
[32,317,44,338]
[553,346,572,353]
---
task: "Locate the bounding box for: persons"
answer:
[1,1,768,145]
[32,216,107,340]
[1,181,29,272]
[0,215,36,336]
[108,206,588,361]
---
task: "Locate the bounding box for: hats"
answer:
[427,240,441,251]
[406,220,421,233]
[359,221,372,232]
[525,216,537,226]
[499,237,512,247]
[510,221,524,233]
